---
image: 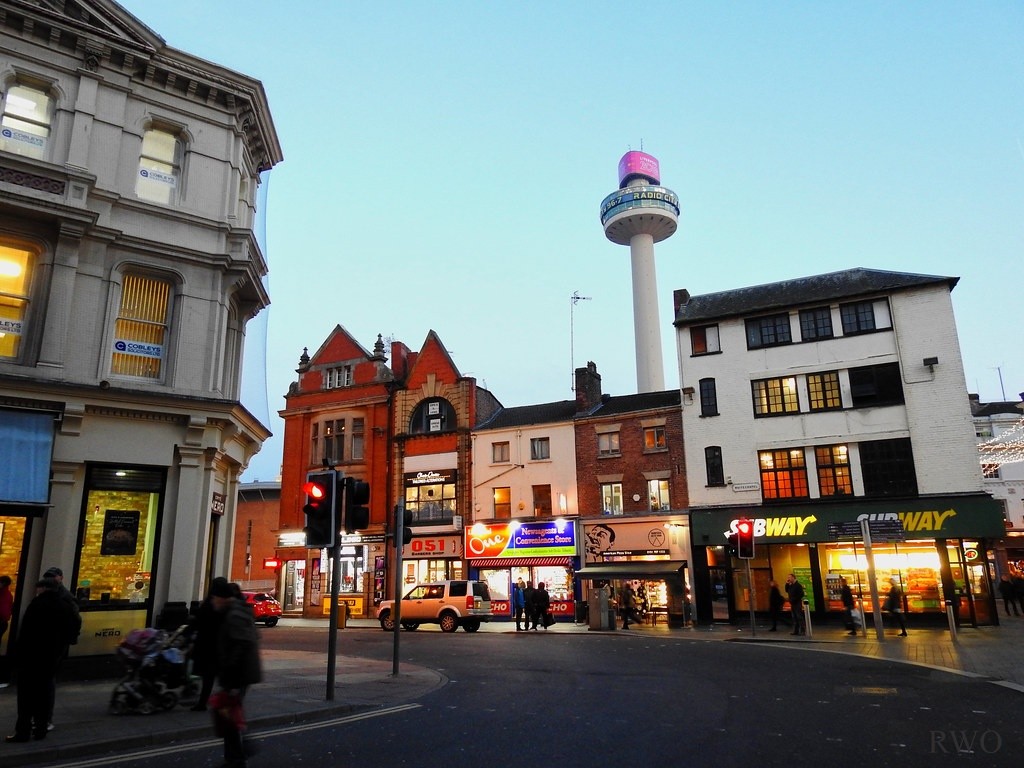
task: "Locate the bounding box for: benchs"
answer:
[645,612,667,624]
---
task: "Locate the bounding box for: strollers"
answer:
[109,624,203,718]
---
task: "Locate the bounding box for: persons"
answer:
[618,584,644,630]
[0,568,82,744]
[651,496,664,510]
[178,577,262,768]
[839,579,857,635]
[514,581,550,631]
[785,574,806,636]
[998,572,1024,617]
[518,577,526,589]
[953,581,961,628]
[886,578,907,637]
[767,581,780,632]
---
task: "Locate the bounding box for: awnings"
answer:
[575,561,687,575]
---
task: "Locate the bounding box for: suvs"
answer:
[376,579,494,633]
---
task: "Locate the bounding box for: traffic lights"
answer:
[725,533,739,559]
[393,505,412,550]
[738,520,755,560]
[345,476,370,535]
[302,469,337,548]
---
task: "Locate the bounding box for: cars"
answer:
[241,591,282,628]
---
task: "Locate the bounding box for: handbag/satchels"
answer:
[544,613,555,626]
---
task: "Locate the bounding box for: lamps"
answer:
[923,356,939,373]
[682,386,695,400]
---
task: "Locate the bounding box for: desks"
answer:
[652,606,672,629]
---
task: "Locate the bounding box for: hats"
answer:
[43,567,63,577]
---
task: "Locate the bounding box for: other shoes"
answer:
[768,627,776,632]
[5,734,21,742]
[790,632,799,635]
[848,632,857,635]
[897,632,907,636]
[46,722,53,733]
[32,731,45,739]
[190,704,207,711]
